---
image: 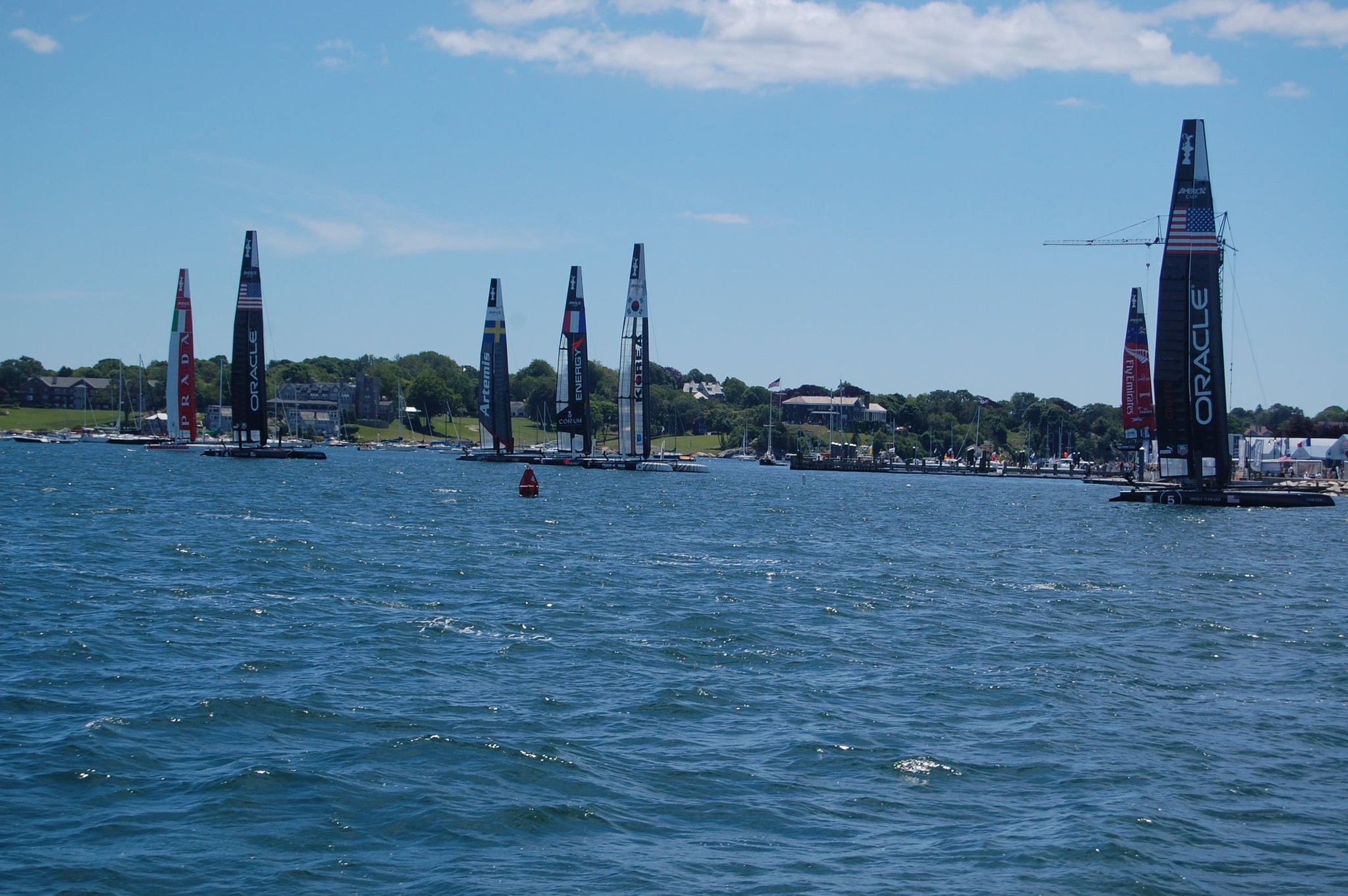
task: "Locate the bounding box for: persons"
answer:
[991,451,1003,463]
[1280,464,1344,479]
[1094,458,1139,474]
[1030,452,1037,463]
[1048,456,1059,468]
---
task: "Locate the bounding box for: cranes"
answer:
[1042,212,1240,324]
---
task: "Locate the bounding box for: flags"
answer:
[768,378,780,388]
[1275,439,1283,448]
[1142,440,1158,464]
[1297,436,1311,447]
[869,445,873,454]
[1068,454,1072,458]
[334,426,339,431]
[1284,437,1291,457]
[1254,440,1263,468]
[1343,439,1348,460]
[1238,439,1246,468]
[934,448,937,454]
[1279,454,1291,461]
[948,448,952,454]
[889,447,895,453]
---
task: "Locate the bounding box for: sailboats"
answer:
[144,268,267,453]
[730,418,757,461]
[758,390,777,466]
[1108,118,1336,508]
[199,230,328,460]
[0,243,712,475]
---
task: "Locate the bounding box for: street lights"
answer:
[1268,436,1278,459]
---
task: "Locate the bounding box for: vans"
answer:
[1056,458,1075,469]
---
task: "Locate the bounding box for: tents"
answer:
[1244,434,1348,477]
